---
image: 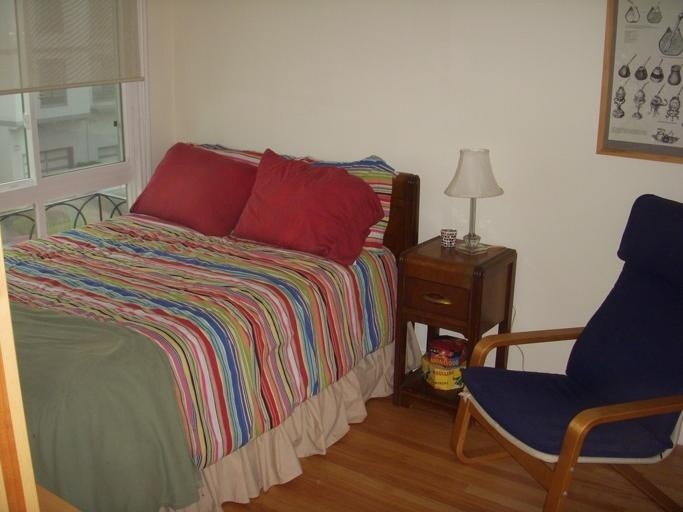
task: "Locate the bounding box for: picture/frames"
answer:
[595,2,683,166]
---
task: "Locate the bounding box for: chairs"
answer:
[451,193,682,509]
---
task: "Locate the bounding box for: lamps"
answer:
[446,147,504,256]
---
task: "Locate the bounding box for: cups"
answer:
[441,229,456,247]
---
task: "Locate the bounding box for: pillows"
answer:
[128,141,384,266]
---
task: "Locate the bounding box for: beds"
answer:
[2,142,420,512]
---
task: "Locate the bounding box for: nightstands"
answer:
[394,237,516,413]
